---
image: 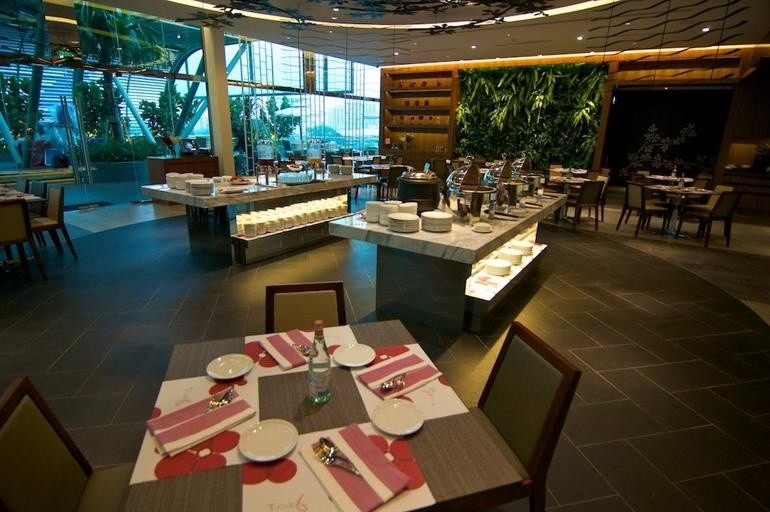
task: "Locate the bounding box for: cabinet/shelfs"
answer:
[378,64,461,172]
[145,155,219,208]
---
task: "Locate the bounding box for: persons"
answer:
[232,128,250,176]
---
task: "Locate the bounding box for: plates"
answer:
[235,197,348,239]
[364,199,454,234]
[332,342,376,368]
[226,179,251,185]
[216,187,248,193]
[238,417,298,463]
[471,228,494,233]
[206,353,254,380]
[371,398,426,437]
[484,238,533,278]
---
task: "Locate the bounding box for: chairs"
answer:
[1,177,81,286]
[262,279,347,332]
[441,316,584,511]
[538,153,746,247]
[277,145,539,200]
[1,373,135,512]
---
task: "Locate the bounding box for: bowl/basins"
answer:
[327,164,341,174]
[473,222,490,230]
[165,172,233,196]
[339,166,354,176]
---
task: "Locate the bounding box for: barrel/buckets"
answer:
[257,140,275,159]
[306,143,322,158]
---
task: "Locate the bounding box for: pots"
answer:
[509,156,548,199]
[394,168,443,216]
[481,158,531,211]
[441,164,500,224]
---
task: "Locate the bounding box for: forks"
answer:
[207,391,225,407]
[313,447,360,477]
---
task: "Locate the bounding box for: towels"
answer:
[353,348,445,403]
[255,326,318,374]
[296,424,414,511]
[143,383,258,461]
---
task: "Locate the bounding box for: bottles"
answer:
[679,172,686,190]
[566,165,572,179]
[307,320,332,405]
[671,165,678,177]
[388,155,393,169]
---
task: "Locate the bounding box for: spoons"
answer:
[319,438,354,466]
[396,373,405,392]
[221,387,233,407]
[380,376,395,394]
